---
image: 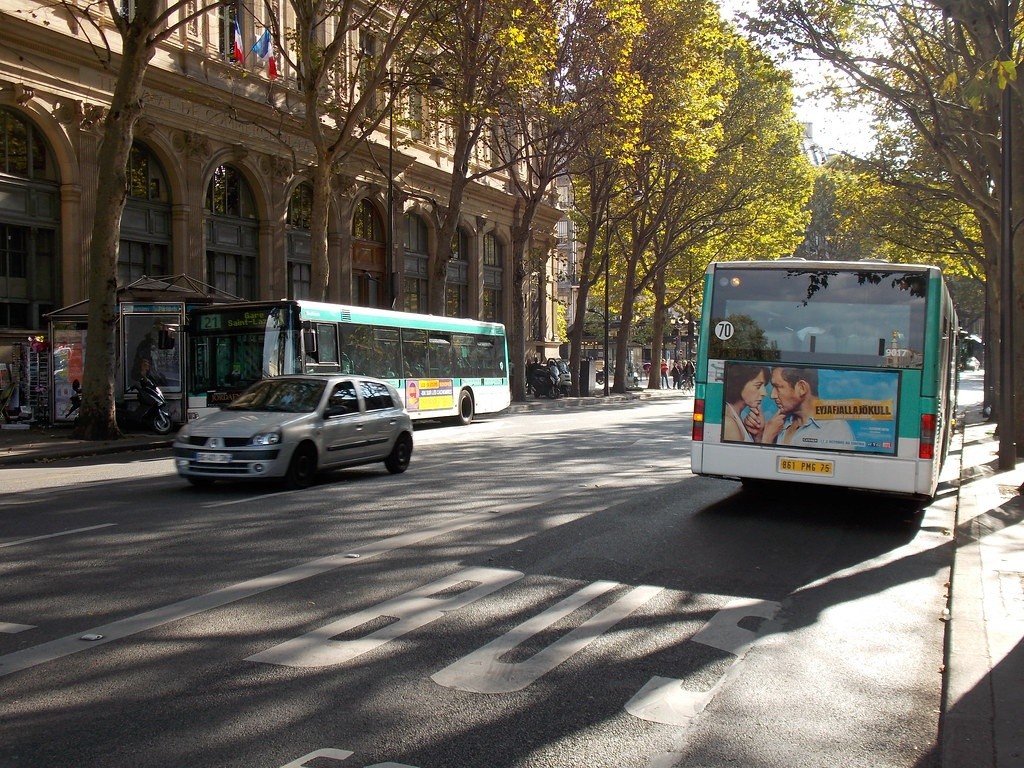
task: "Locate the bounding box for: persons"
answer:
[241,335,502,378]
[743,364,857,451]
[509,355,697,395]
[129,316,180,388]
[280,382,316,412]
[723,362,773,444]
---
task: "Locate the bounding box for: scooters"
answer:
[546,358,573,397]
[595,367,605,385]
[64,369,173,435]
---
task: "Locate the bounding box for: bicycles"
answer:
[681,374,693,395]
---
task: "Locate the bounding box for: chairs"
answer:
[759,329,889,356]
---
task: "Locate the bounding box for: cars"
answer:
[175,374,412,487]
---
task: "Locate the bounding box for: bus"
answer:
[690,260,967,500]
[166,297,510,426]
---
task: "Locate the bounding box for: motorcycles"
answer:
[530,365,560,399]
